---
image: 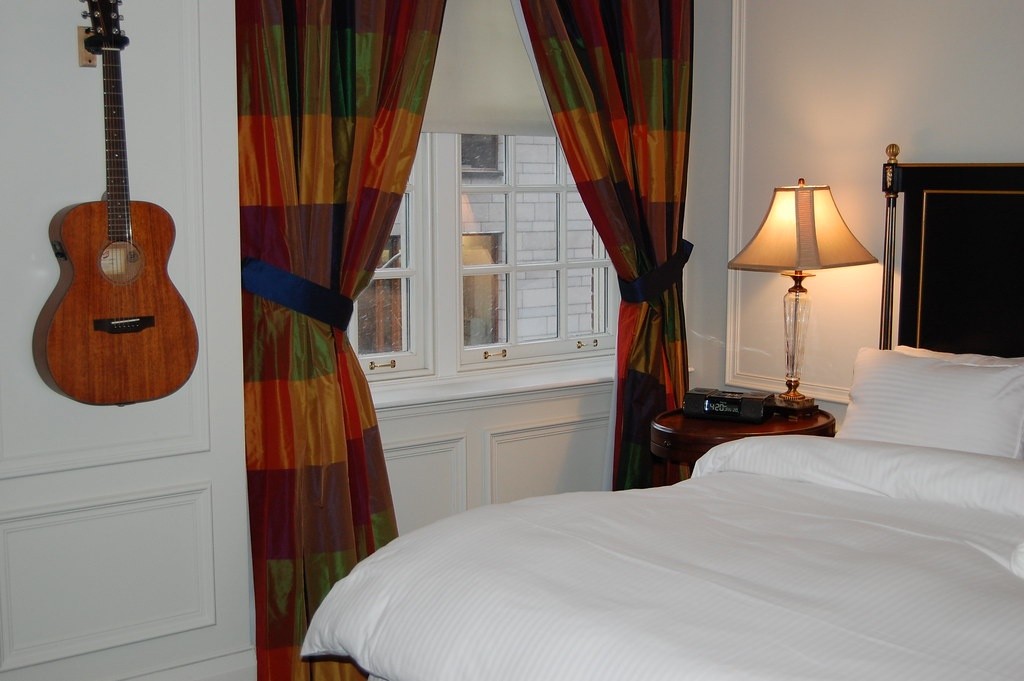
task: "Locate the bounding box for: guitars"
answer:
[30,0,201,408]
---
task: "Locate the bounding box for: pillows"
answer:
[834,346,1024,459]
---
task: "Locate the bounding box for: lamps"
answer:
[726,178,878,419]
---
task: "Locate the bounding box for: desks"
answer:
[650,406,836,462]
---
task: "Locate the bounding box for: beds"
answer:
[299,143,1024,681]
